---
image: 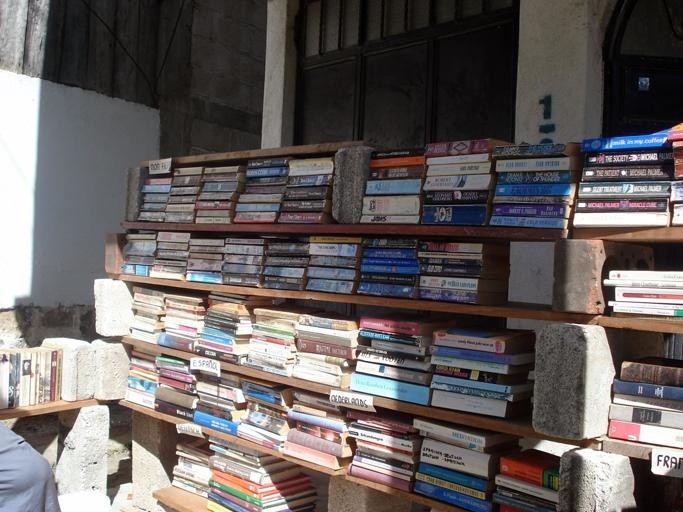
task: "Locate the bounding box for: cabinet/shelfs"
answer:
[109,142,683,512]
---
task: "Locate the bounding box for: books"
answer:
[116,121,682,512]
[1,345,62,410]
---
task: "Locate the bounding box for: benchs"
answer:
[0,399,102,422]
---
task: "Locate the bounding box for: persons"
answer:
[0,418,62,512]
